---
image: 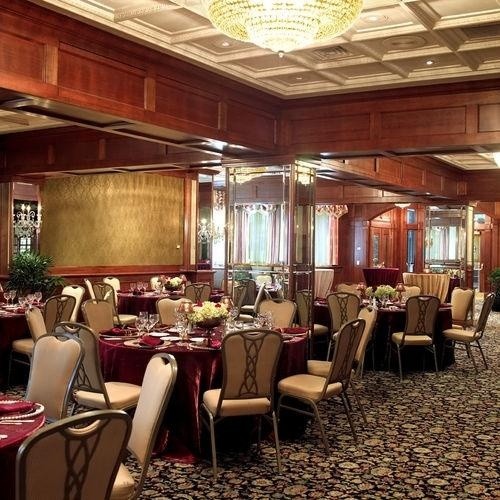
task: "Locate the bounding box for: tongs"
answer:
[10,291,16,306]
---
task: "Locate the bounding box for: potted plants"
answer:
[488,267,500,312]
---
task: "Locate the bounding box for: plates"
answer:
[160,337,181,341]
[189,343,221,350]
[148,332,169,337]
[0,400,44,419]
[281,333,305,336]
[190,337,207,341]
[168,329,189,332]
[98,332,142,337]
[127,327,136,329]
[124,340,171,348]
[1,306,14,309]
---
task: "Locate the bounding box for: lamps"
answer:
[208,0,366,57]
[13,203,42,240]
[196,218,222,244]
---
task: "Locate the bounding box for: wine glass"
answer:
[229,307,238,320]
[139,312,148,327]
[144,319,153,333]
[254,318,264,328]
[35,292,42,306]
[27,294,35,308]
[236,321,244,329]
[149,314,159,332]
[135,318,145,336]
[18,295,27,312]
[142,283,148,292]
[4,293,11,306]
[130,283,136,291]
[267,319,275,329]
[137,282,142,291]
[229,321,235,329]
